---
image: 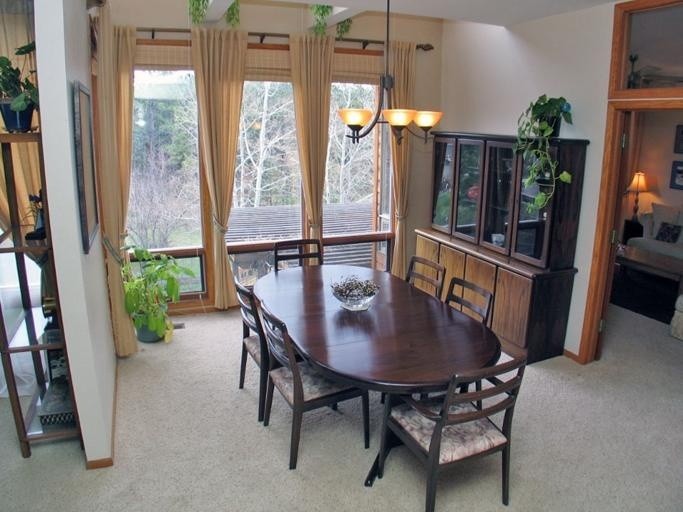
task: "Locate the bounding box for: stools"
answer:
[670,293,683,340]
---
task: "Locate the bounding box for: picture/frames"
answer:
[669,161,683,189]
[673,125,683,153]
[72,80,99,253]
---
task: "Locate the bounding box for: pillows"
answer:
[655,222,683,244]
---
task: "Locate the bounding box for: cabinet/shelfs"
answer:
[413,228,580,363]
[0,132,78,458]
[429,133,588,270]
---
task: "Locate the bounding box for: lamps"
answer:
[336,1,443,144]
[626,171,648,221]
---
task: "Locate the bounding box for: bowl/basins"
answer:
[332,276,380,312]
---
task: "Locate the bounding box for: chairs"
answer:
[274,239,322,271]
[379,352,528,511]
[404,256,447,298]
[258,299,369,470]
[421,276,492,412]
[234,275,330,423]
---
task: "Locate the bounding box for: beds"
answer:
[608,202,683,324]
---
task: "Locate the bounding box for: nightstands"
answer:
[622,219,643,244]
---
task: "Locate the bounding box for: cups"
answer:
[491,233,504,246]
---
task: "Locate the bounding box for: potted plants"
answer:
[0,40,38,133]
[121,248,196,343]
[514,95,573,209]
